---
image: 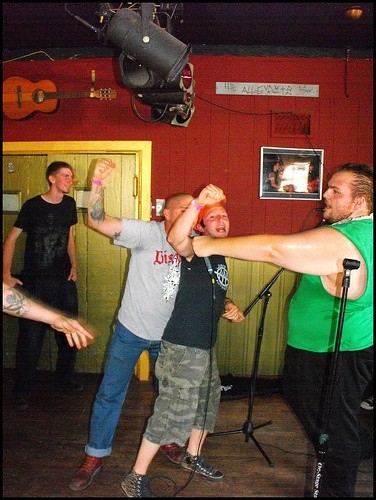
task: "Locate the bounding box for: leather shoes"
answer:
[69,457,105,492]
[161,441,186,464]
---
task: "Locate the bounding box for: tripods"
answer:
[206,267,285,467]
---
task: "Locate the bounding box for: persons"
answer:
[118,181,240,498]
[1,281,94,350]
[67,157,246,492]
[263,161,319,193]
[190,161,373,498]
[2,161,85,408]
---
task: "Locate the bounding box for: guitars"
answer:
[3,76,117,120]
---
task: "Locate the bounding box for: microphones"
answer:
[316,208,324,212]
[193,231,213,275]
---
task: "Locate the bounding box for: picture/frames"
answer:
[74,187,93,213]
[3,190,22,215]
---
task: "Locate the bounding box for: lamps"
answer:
[64,0,197,127]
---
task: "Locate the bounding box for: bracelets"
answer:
[191,198,204,211]
[88,177,105,186]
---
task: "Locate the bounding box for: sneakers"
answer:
[360,396,373,410]
[53,377,84,393]
[121,470,156,497]
[14,393,29,412]
[180,454,225,482]
